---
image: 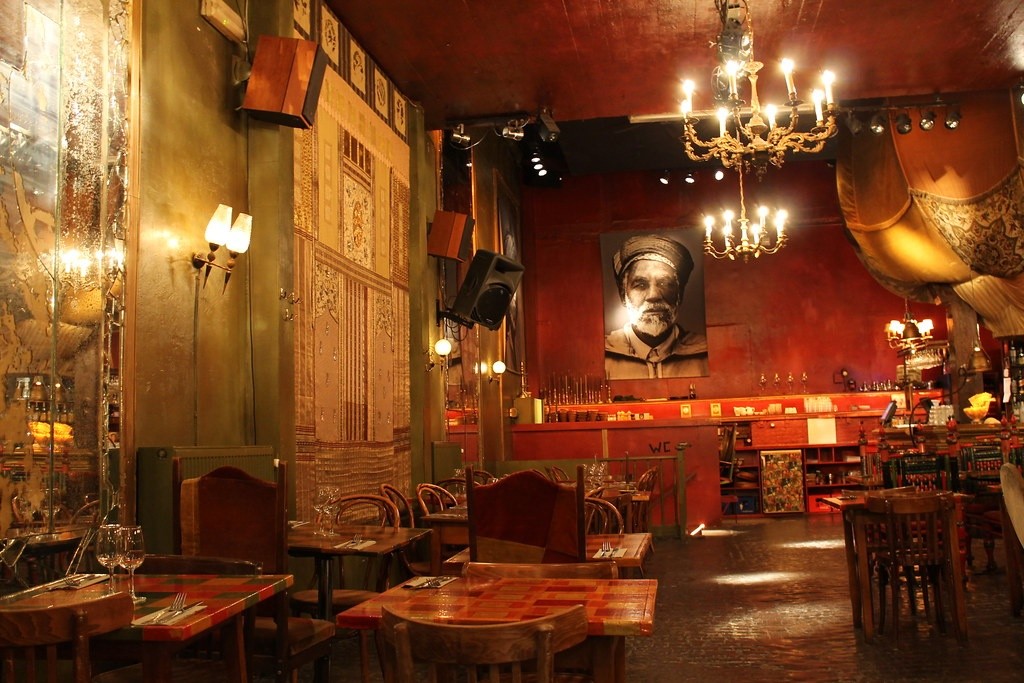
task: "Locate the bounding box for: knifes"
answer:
[414,574,444,590]
[157,600,203,625]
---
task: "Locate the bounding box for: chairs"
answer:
[840,488,969,651]
[1,451,657,683]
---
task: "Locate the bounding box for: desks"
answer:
[418,499,469,577]
[335,575,659,683]
[286,519,434,683]
[441,530,652,570]
[0,574,294,683]
[821,493,976,642]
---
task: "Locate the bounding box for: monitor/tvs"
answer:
[880,400,897,428]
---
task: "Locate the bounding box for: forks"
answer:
[424,577,451,587]
[150,592,187,625]
[62,575,95,586]
[339,533,363,551]
[598,541,610,558]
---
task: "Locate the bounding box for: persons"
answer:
[604,233,710,379]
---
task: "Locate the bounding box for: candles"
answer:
[538,371,611,395]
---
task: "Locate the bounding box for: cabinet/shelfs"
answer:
[718,417,864,521]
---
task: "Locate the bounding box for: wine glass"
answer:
[96,525,123,597]
[116,525,147,603]
[313,484,341,538]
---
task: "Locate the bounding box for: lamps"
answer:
[966,322,992,373]
[489,360,506,385]
[189,203,252,297]
[451,123,470,147]
[843,107,961,135]
[885,293,934,350]
[660,168,724,185]
[530,140,548,177]
[536,114,561,142]
[429,338,452,375]
[503,120,525,141]
[677,13,841,265]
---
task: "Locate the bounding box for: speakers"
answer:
[242,34,328,129]
[452,249,527,331]
[426,210,476,263]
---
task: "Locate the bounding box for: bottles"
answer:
[689,381,696,400]
